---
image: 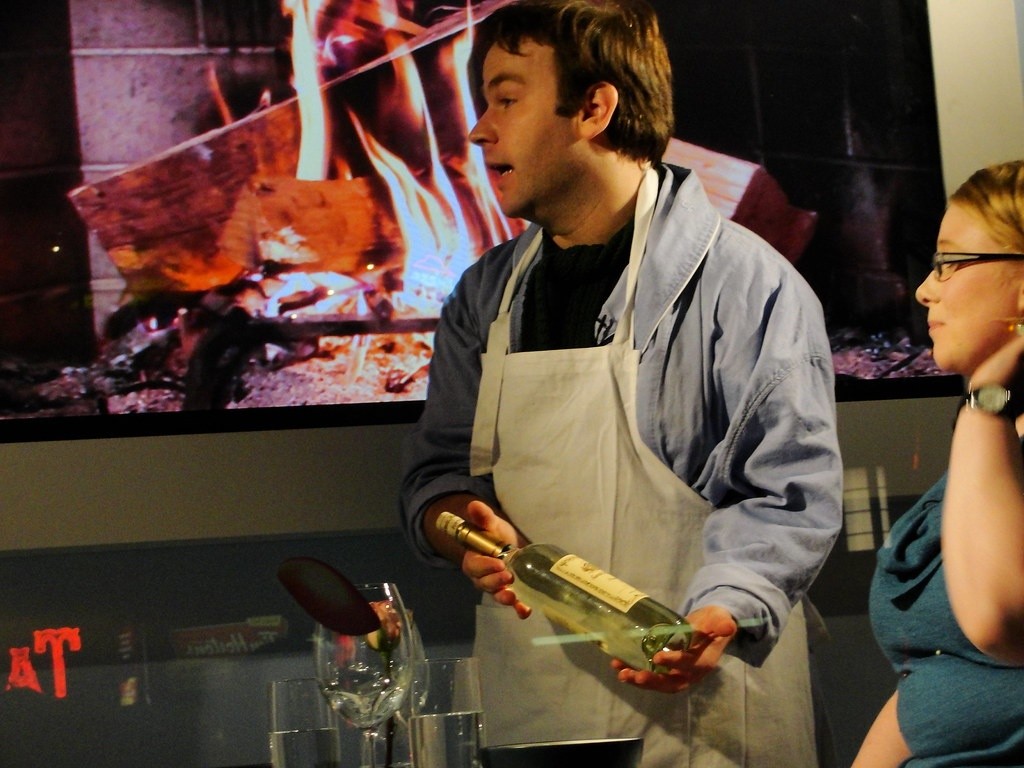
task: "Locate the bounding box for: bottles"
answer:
[435,511,693,675]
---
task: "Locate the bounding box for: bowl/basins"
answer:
[481,737,646,768]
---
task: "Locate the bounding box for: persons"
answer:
[399,1,843,768]
[852,158,1023,768]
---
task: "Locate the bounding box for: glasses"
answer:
[933,250,1024,281]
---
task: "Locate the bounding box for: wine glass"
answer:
[317,583,414,767]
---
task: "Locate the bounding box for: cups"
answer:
[409,657,531,768]
[269,675,341,768]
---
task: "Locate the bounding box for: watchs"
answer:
[953,385,1015,430]
